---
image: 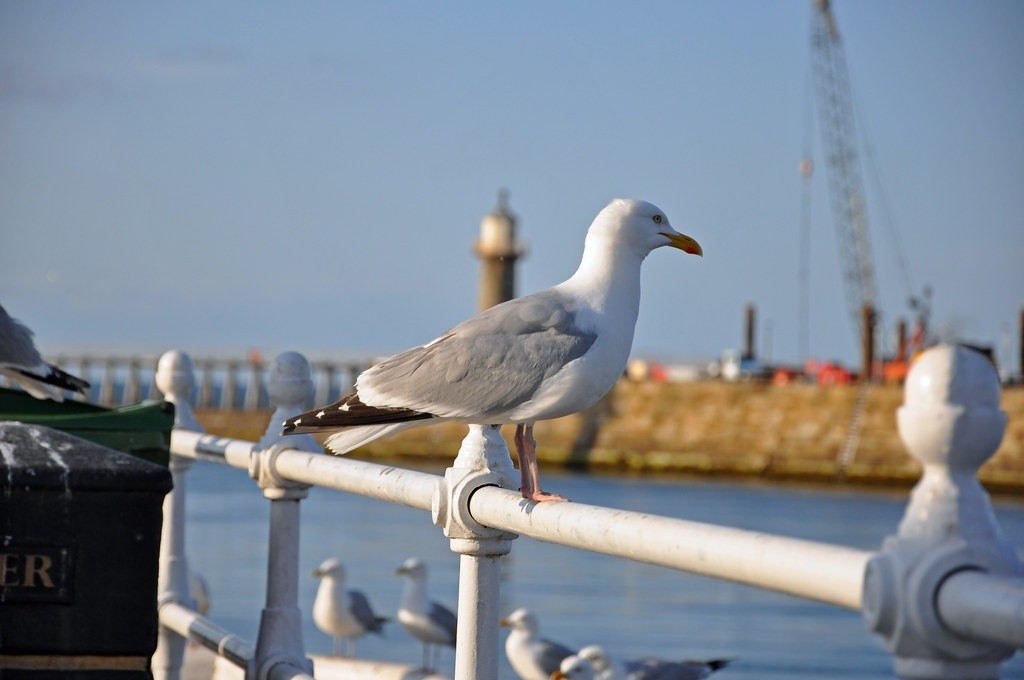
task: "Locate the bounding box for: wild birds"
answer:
[279,198,704,503]
[0,294,92,394]
[500,606,739,680]
[393,557,457,673]
[309,559,390,657]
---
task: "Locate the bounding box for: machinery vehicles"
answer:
[802,0,931,388]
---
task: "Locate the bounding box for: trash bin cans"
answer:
[0,421,176,680]
[0,400,176,468]
